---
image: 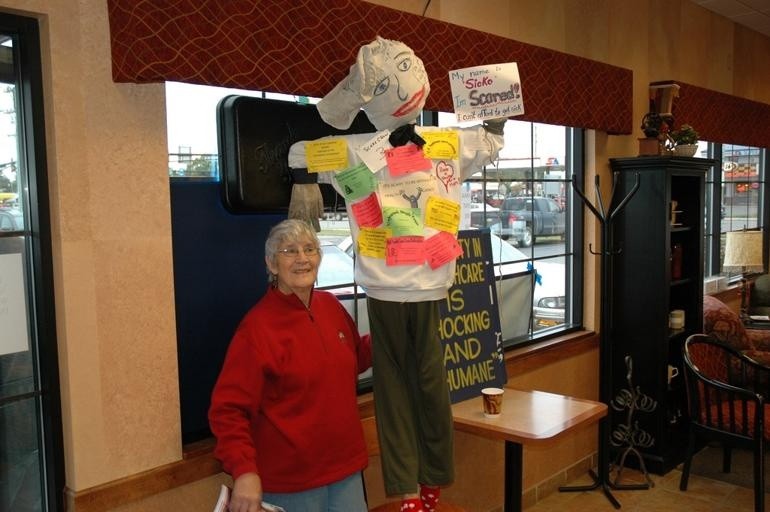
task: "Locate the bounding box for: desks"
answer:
[452,386,609,512]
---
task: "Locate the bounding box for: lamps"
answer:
[723,225,764,323]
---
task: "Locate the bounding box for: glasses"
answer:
[278,247,321,257]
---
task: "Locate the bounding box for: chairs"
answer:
[679,333,770,512]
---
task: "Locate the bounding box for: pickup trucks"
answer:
[471,196,565,248]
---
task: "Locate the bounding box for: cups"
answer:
[670,310,685,329]
[481,388,504,419]
[668,366,679,384]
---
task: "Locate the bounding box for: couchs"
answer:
[702,295,769,382]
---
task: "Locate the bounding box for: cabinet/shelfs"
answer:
[598,154,720,480]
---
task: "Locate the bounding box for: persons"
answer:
[208,220,374,512]
[286,34,506,512]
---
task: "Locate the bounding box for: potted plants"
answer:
[671,122,699,157]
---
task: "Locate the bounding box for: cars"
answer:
[0,210,26,254]
